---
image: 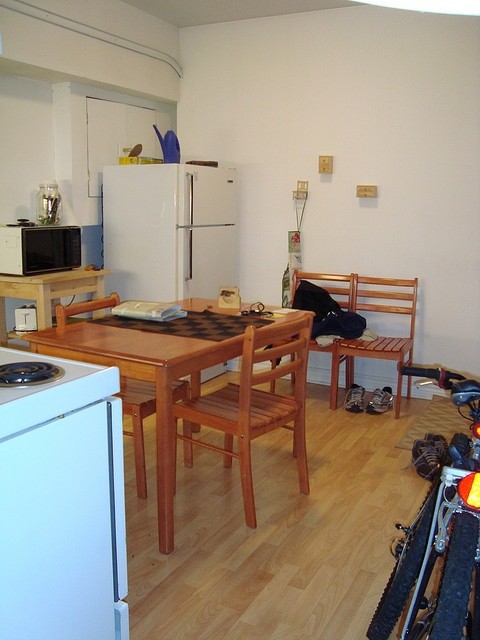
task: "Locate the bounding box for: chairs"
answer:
[55,292,193,499]
[330,274,420,418]
[269,271,354,413]
[171,314,314,529]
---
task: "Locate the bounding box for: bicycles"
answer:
[365,364,480,638]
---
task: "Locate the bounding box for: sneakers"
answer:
[366,387,393,414]
[344,383,365,413]
[400,439,442,481]
[425,432,453,466]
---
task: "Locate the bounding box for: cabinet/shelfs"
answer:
[1,267,114,351]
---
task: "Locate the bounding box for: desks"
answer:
[25,296,316,554]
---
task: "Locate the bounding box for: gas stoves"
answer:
[0,344,122,442]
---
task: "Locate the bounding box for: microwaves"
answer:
[0,224,82,276]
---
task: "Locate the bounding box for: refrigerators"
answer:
[103,163,239,309]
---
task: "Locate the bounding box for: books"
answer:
[111,300,187,321]
